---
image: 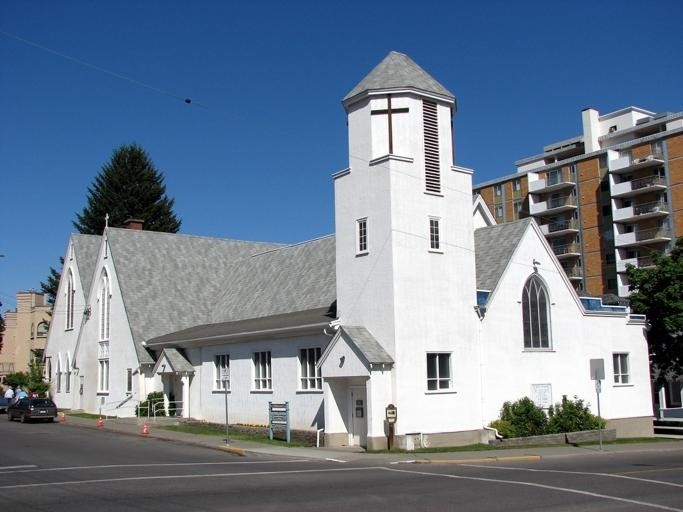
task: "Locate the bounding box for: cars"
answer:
[6,396,56,423]
[0,396,12,414]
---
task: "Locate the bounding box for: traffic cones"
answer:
[140,420,149,434]
[97,415,104,427]
[58,412,65,421]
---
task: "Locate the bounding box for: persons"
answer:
[3,386,14,414]
[18,390,28,400]
[15,385,21,395]
[29,390,38,398]
